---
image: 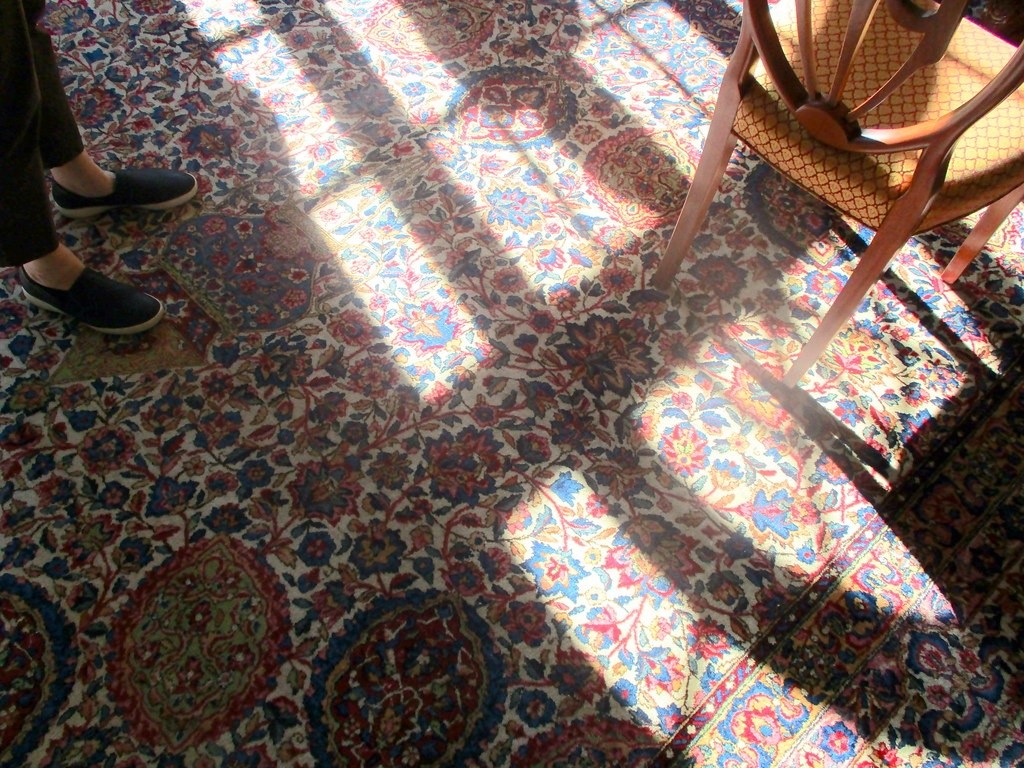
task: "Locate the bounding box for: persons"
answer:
[0,0,197,335]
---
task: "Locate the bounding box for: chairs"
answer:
[651,0,1024,389]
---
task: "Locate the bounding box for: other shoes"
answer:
[18,265,164,335]
[49,168,198,218]
